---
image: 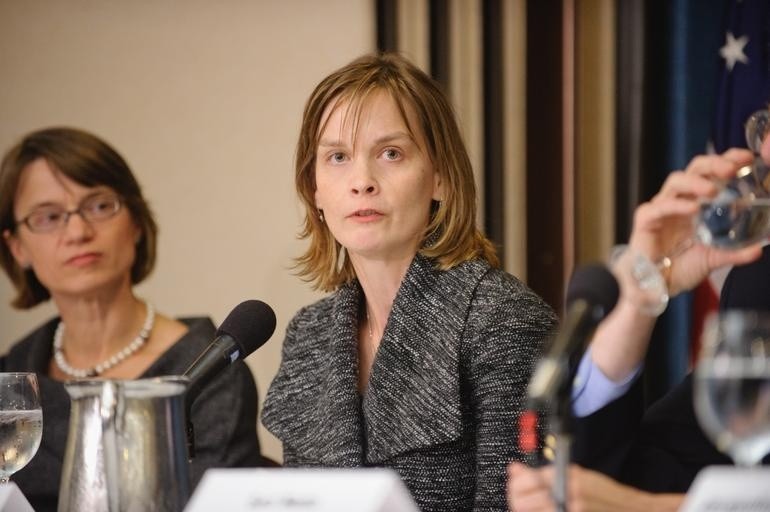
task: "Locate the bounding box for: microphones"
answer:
[524,262,621,413]
[174,299,277,412]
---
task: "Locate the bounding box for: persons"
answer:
[260,54,559,512]
[507,149,770,512]
[1,128,258,512]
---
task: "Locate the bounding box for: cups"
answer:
[0,371,43,482]
[693,309,769,470]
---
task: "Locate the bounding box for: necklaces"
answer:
[52,300,156,379]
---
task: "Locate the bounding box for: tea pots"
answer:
[58,375,189,512]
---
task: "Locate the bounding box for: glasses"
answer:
[15,192,126,234]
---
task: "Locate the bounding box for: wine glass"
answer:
[609,158,769,315]
